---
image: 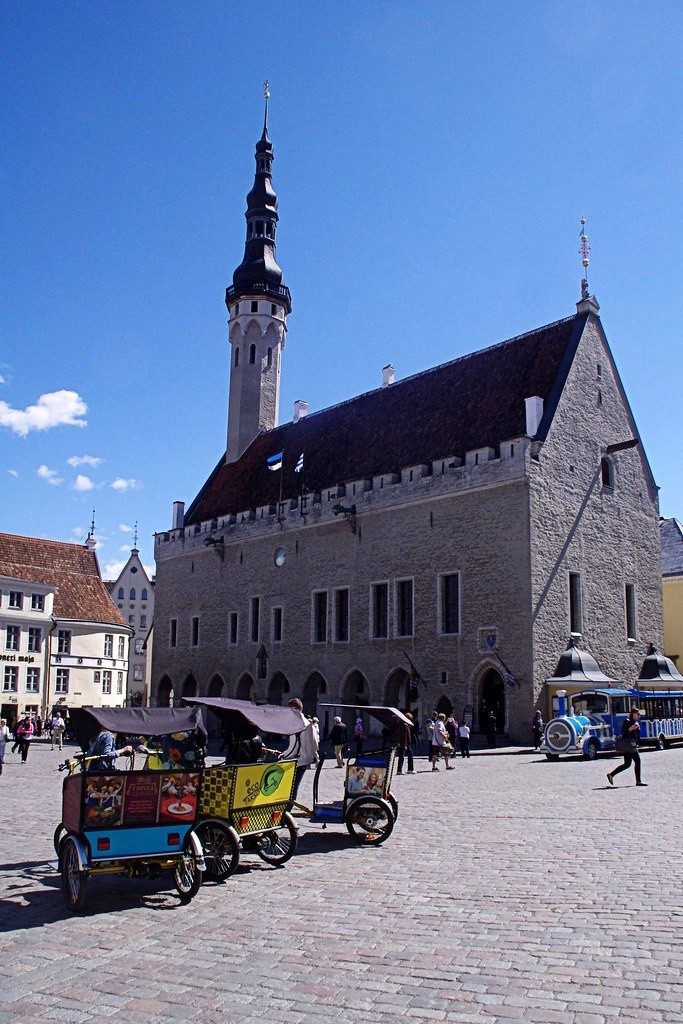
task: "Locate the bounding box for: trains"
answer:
[538,689,683,761]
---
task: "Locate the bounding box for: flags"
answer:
[295,453,303,472]
[267,450,283,471]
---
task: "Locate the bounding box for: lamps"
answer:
[203,536,224,546]
[332,504,357,533]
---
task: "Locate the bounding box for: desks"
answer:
[160,795,197,823]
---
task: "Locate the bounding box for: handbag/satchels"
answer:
[439,742,455,755]
[616,737,637,753]
[4,735,10,742]
[20,722,25,737]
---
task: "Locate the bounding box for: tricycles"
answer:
[180,695,315,883]
[52,705,209,911]
[292,702,417,846]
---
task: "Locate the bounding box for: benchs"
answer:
[347,741,399,795]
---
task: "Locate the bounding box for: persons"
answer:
[588,700,605,714]
[0,712,265,775]
[84,782,122,824]
[277,698,317,812]
[531,710,544,749]
[160,778,199,799]
[346,767,384,806]
[302,697,505,769]
[606,708,648,786]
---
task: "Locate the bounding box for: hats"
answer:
[357,718,362,723]
[426,719,433,724]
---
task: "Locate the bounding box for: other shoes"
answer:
[397,771,404,775]
[11,748,14,753]
[432,767,439,772]
[408,770,416,774]
[21,760,26,764]
[636,782,647,786]
[446,766,456,769]
[336,763,345,769]
[607,774,614,785]
